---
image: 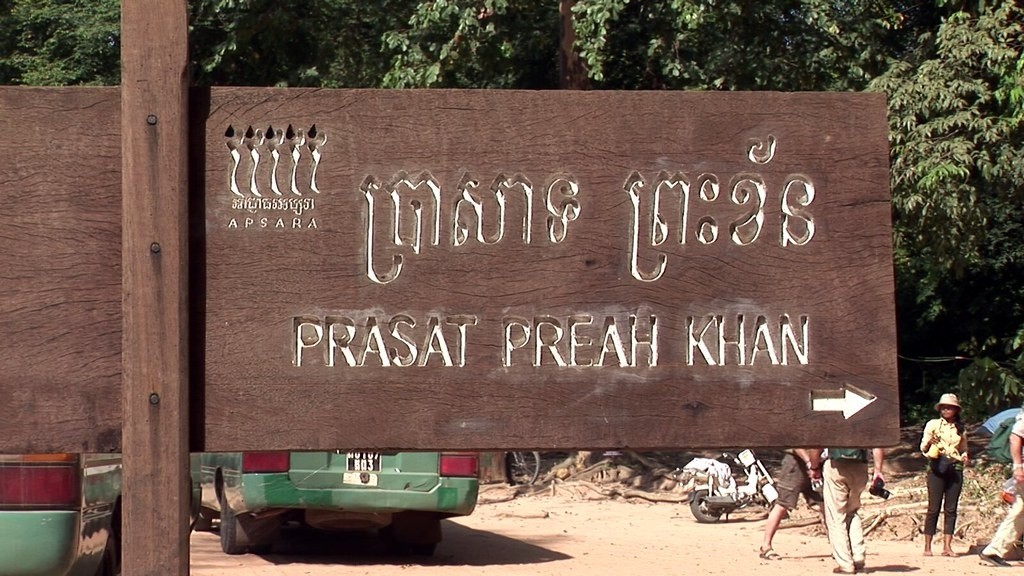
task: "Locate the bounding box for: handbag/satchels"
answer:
[929,457,953,478]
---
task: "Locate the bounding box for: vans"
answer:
[187,449,483,558]
[0,452,122,576]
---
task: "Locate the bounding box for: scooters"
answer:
[677,446,791,525]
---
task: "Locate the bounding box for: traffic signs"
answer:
[196,94,901,448]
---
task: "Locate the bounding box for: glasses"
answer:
[939,406,954,411]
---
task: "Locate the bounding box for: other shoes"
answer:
[833,563,865,574]
[978,554,1011,567]
[924,550,932,556]
[941,550,959,557]
[759,546,782,560]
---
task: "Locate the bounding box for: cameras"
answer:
[869,477,890,499]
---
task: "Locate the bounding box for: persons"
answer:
[810,446,885,574]
[761,448,828,562]
[980,415,1024,568]
[921,393,969,556]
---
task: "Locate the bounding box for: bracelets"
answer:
[810,467,821,471]
[1013,464,1023,470]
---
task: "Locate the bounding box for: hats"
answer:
[933,394,962,413]
